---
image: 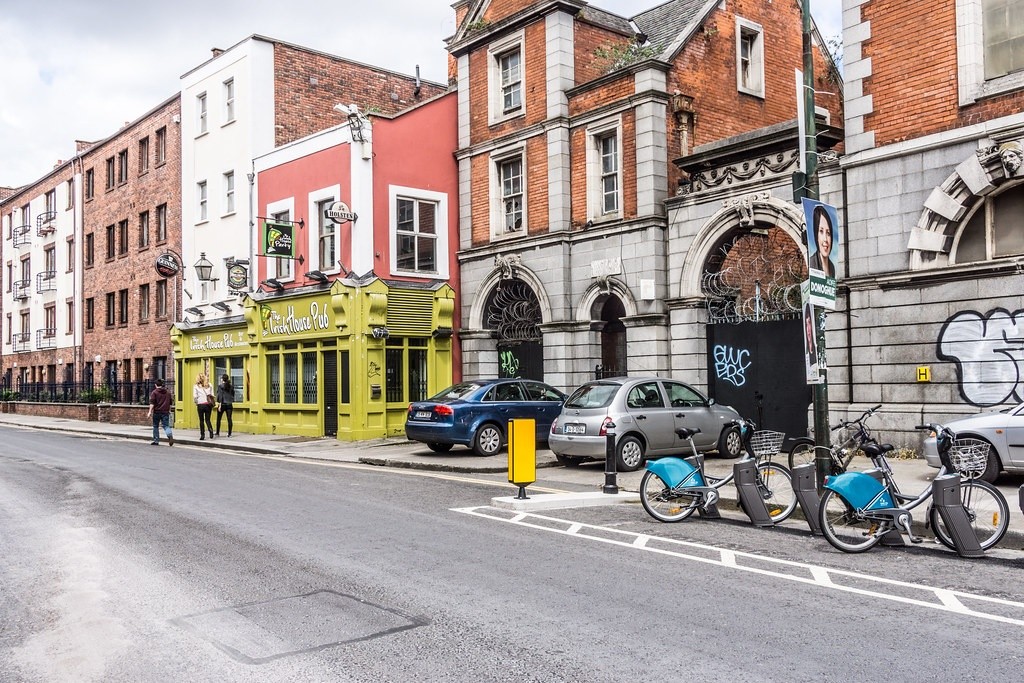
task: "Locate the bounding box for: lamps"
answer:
[302,270,327,281]
[372,326,389,339]
[192,251,220,282]
[210,301,233,312]
[184,307,206,317]
[260,278,284,291]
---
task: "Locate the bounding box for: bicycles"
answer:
[818,423,1010,553]
[640,419,799,524]
[788,404,904,505]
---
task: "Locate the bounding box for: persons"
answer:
[213,374,235,437]
[192,372,213,440]
[147,379,174,446]
[805,303,817,366]
[809,206,836,277]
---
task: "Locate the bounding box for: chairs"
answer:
[506,388,521,401]
[646,390,660,407]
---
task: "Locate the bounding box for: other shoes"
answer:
[227,434,232,438]
[213,433,219,436]
[210,428,213,439]
[169,436,173,446]
[200,435,205,440]
[151,441,158,445]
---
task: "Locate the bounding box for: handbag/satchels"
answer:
[207,394,216,407]
[217,402,222,412]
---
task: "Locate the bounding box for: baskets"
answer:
[948,438,991,471]
[750,430,785,454]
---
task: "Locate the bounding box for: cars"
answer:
[923,403,1024,485]
[405,378,570,457]
[548,376,747,472]
